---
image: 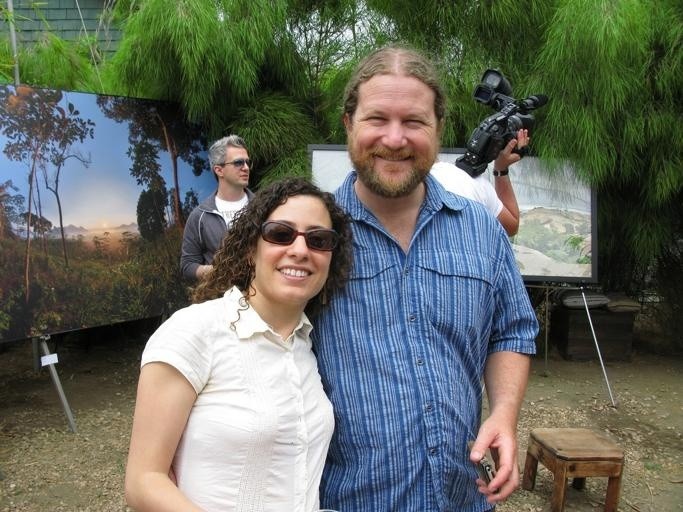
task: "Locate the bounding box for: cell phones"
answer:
[465,440,499,496]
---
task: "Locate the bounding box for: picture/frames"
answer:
[306,143,601,286]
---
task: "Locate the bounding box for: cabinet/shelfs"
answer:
[557,308,637,363]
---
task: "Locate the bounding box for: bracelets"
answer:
[492,168,509,177]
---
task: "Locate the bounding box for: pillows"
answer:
[547,289,641,313]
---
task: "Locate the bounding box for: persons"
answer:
[430,128,530,236]
[307,46,539,512]
[123,178,358,512]
[177,133,261,289]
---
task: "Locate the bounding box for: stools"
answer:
[523,427,626,510]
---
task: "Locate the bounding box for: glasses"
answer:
[261,220,341,252]
[221,159,254,170]
